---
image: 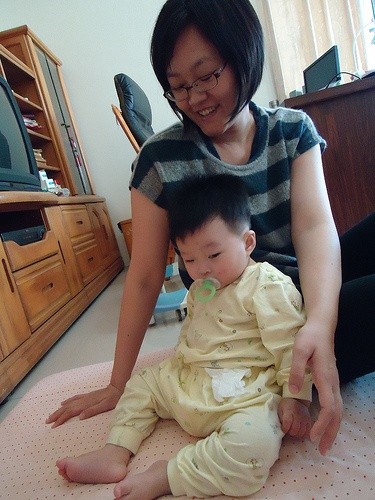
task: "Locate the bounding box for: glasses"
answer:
[163,63,227,101]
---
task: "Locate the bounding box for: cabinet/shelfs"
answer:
[0,24,125,404]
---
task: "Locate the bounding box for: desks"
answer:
[279,76,375,237]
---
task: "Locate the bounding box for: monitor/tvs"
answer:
[303,45,341,94]
[0,74,42,192]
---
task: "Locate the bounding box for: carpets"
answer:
[0,347,375,500]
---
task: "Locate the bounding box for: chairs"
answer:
[110,73,155,154]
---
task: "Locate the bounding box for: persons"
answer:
[46,1,375,456]
[56,174,314,500]
